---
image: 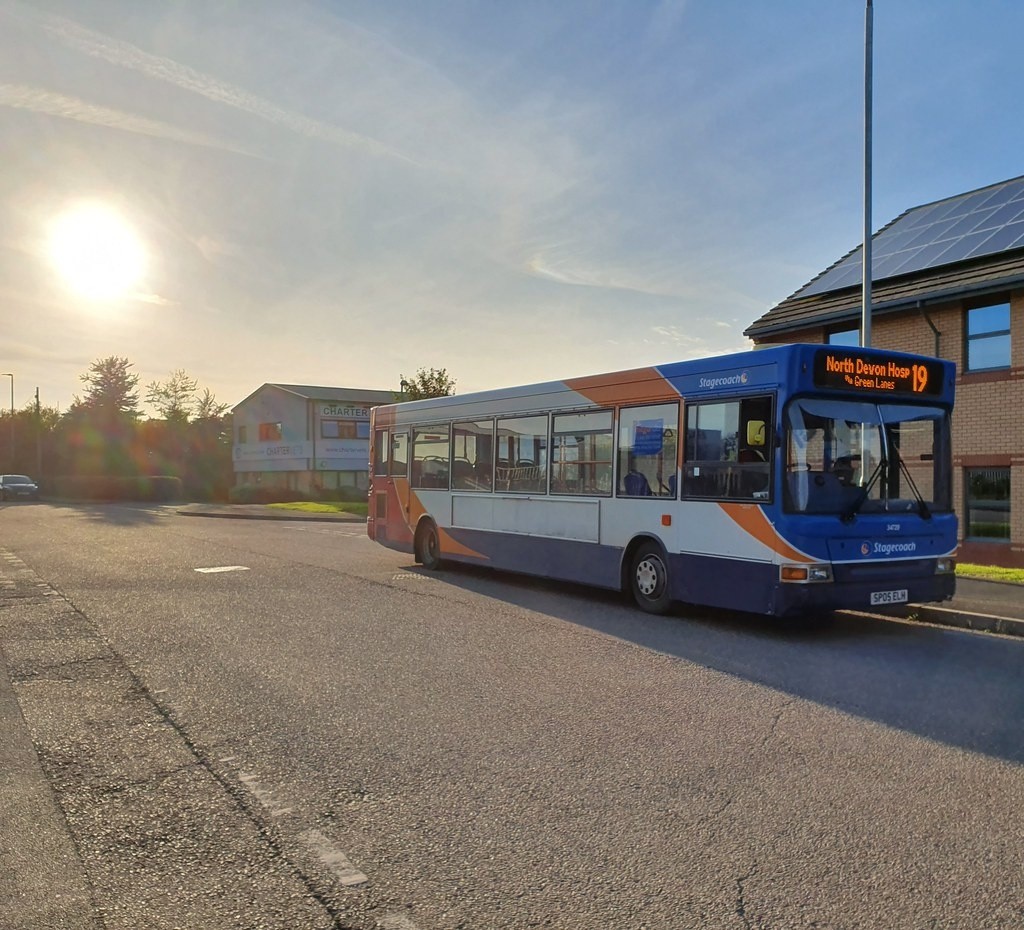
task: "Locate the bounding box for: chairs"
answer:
[386,456,675,497]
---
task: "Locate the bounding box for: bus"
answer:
[361,341,962,623]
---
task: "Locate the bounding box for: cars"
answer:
[0,474,39,502]
